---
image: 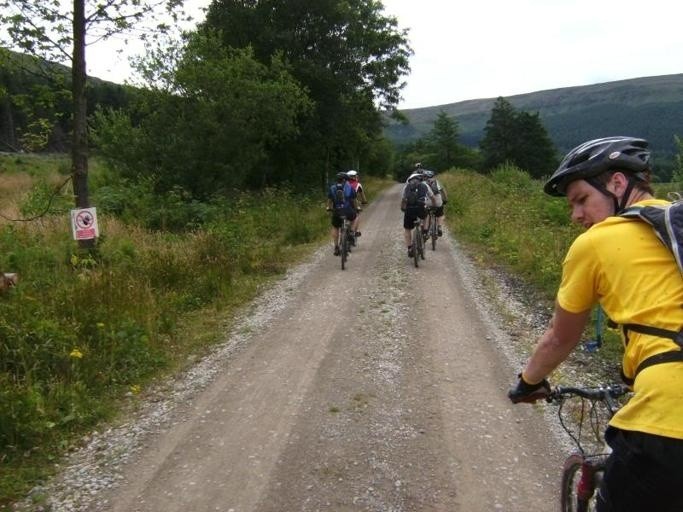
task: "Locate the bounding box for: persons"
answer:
[344,169,367,238]
[423,169,450,238]
[398,174,428,258]
[500,135,682,512]
[415,171,435,240]
[411,163,429,176]
[323,172,359,257]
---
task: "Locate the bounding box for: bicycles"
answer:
[407,198,443,270]
[322,196,366,269]
[510,385,632,511]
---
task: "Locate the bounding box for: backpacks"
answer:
[332,189,346,209]
[406,190,421,206]
[624,199,683,280]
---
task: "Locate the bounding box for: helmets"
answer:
[407,162,435,184]
[542,134,651,196]
[336,170,359,182]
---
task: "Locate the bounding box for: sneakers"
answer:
[404,226,443,258]
[332,229,362,256]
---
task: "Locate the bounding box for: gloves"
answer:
[517,374,551,400]
